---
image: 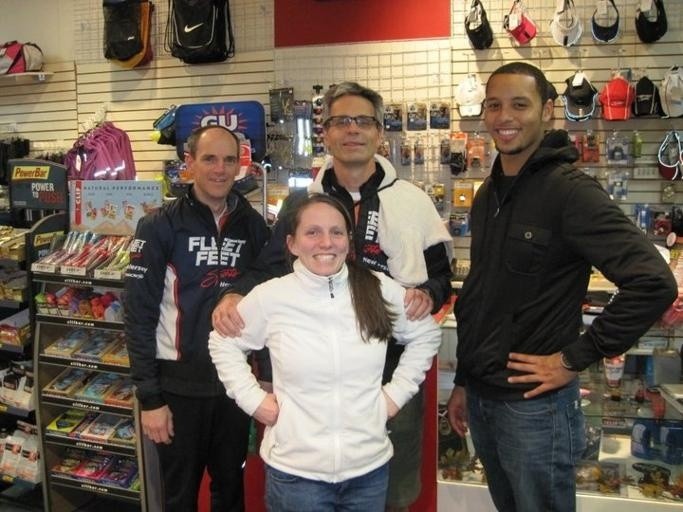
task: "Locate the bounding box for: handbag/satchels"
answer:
[0,41,43,75]
[99,0,153,69]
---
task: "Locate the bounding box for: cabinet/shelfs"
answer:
[0,209,163,511]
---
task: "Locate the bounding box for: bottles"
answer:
[603,355,625,388]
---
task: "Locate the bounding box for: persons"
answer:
[119,82,456,511]
[446,62,678,512]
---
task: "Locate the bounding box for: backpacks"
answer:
[168,0,235,64]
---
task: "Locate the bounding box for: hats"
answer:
[504,1,537,44]
[550,0,584,48]
[658,65,683,117]
[631,77,662,117]
[455,77,484,118]
[656,131,682,181]
[590,1,621,45]
[635,1,667,45]
[598,79,633,121]
[560,74,597,123]
[465,2,493,50]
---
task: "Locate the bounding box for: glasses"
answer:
[322,115,381,129]
